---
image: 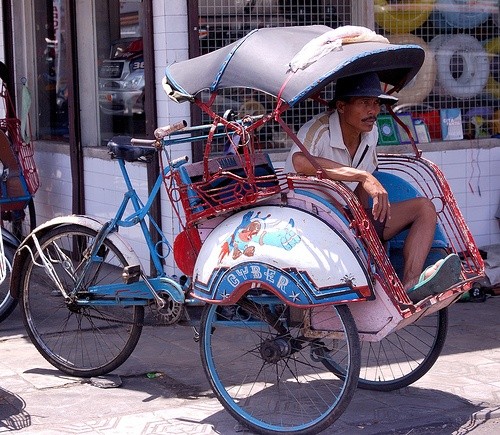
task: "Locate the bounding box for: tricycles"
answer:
[9,23,486,435]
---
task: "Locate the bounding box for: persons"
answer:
[284,71,462,304]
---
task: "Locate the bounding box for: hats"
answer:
[328,71,399,109]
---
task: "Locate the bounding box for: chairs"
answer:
[178,152,450,341]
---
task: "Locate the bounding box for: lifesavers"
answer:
[435,0,494,27]
[379,32,437,106]
[428,33,500,100]
[373,1,433,33]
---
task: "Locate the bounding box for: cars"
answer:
[97,51,145,118]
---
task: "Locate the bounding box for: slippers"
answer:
[405,253,461,304]
